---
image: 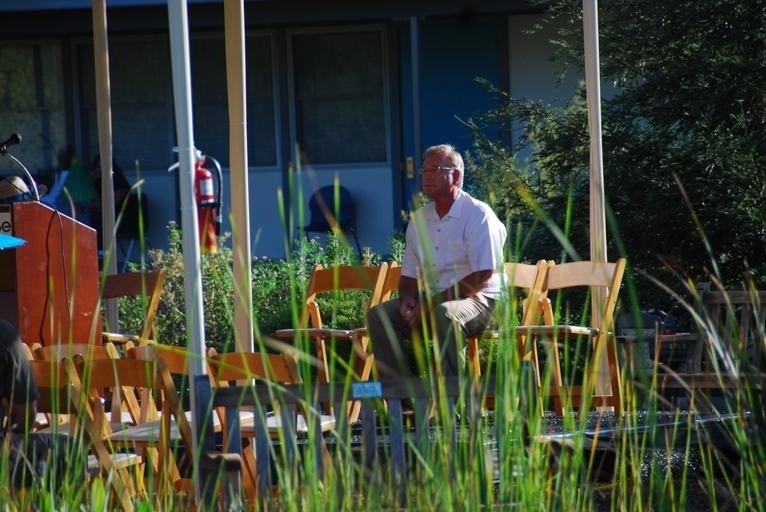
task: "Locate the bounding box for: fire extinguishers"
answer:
[169,146,221,254]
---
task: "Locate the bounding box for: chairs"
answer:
[651,283,766,389]
[516,256,628,419]
[468,259,546,415]
[119,192,150,273]
[297,184,362,258]
[273,261,388,413]
[348,260,406,421]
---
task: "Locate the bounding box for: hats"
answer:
[0,176,48,208]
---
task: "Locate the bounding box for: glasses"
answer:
[418,166,451,175]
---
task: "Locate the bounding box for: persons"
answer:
[0,320,86,506]
[371,145,507,428]
